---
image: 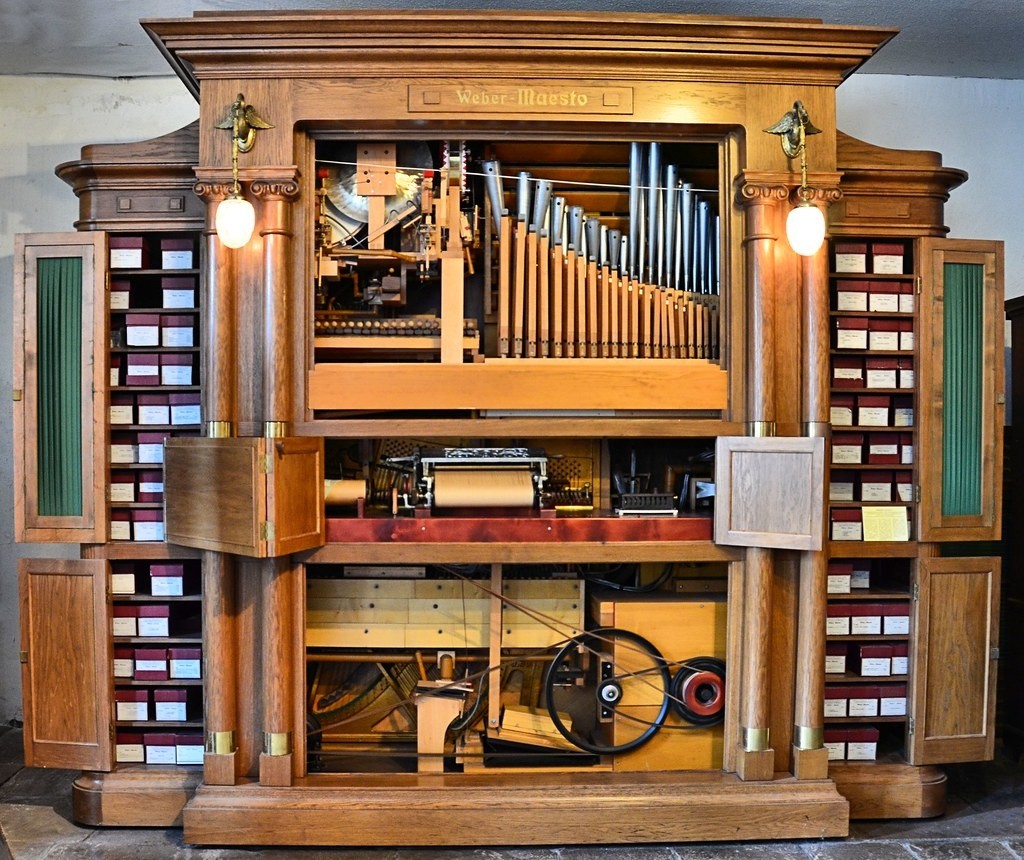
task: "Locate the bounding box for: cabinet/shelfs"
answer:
[11,6,1009,846]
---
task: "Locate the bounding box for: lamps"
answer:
[211,92,276,248]
[760,100,826,257]
